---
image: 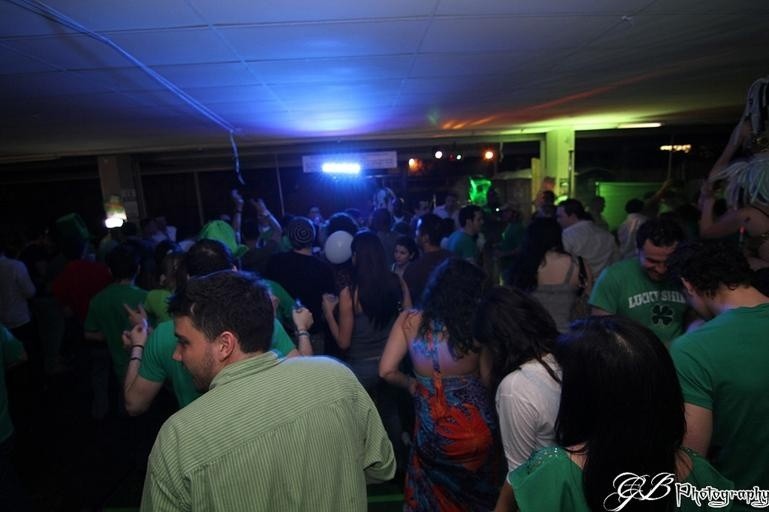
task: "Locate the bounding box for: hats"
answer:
[325,230,354,264]
[288,217,316,247]
[198,219,250,262]
